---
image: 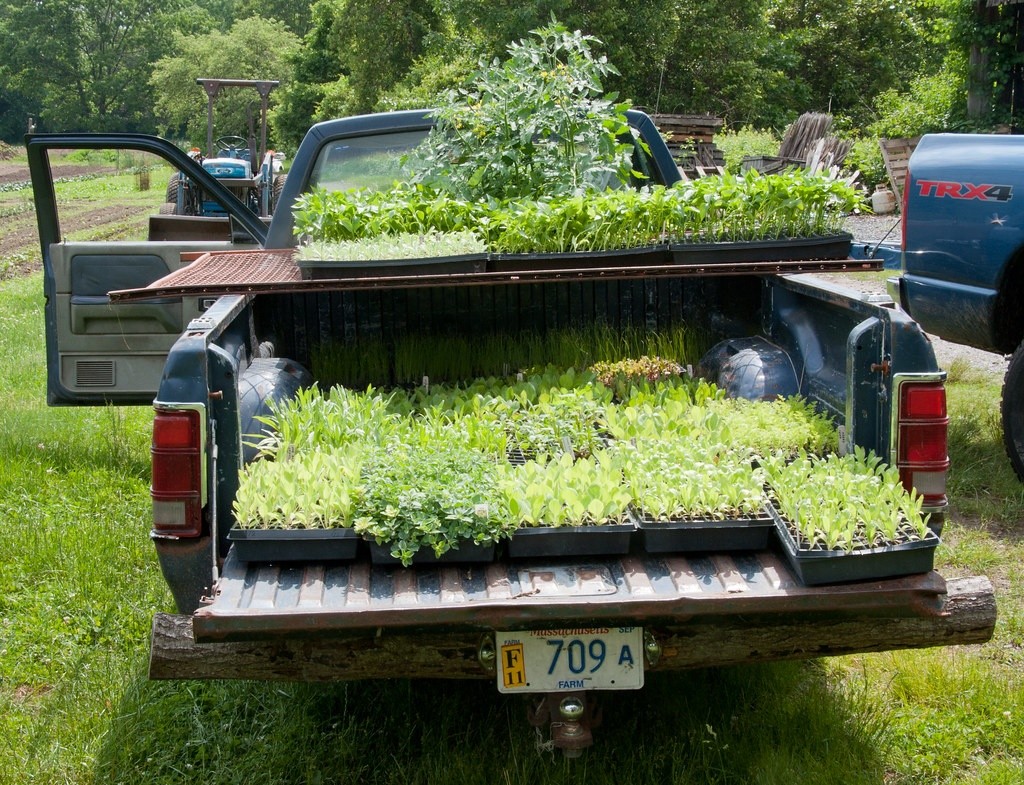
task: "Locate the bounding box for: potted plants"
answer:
[220,313,940,589]
[287,9,899,279]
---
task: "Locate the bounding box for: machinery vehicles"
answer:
[148,76,287,241]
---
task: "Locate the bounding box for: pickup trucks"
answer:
[882,131,1024,481]
[21,99,954,761]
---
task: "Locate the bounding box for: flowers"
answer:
[588,354,689,386]
